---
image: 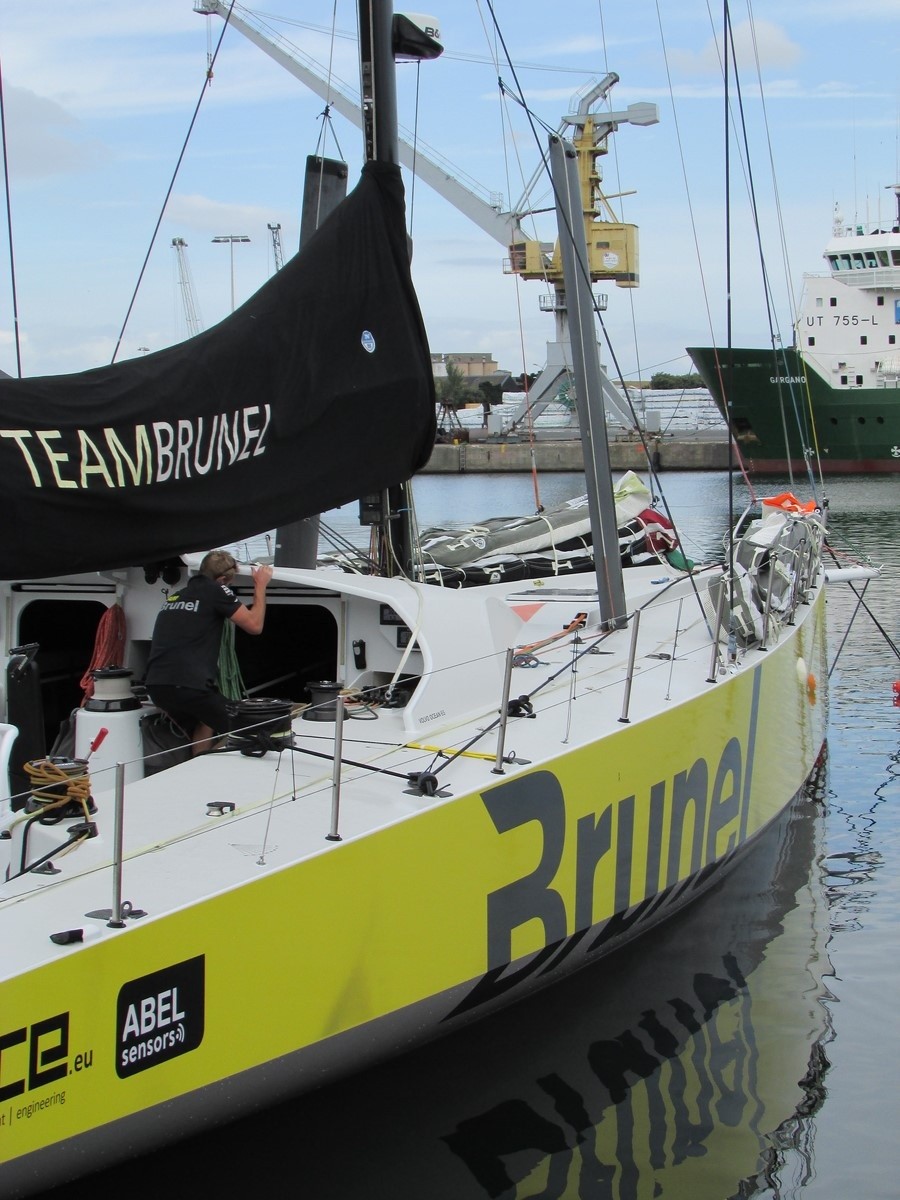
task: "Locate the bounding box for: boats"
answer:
[0,0,832,1200]
[685,137,900,475]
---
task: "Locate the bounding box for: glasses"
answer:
[214,557,238,580]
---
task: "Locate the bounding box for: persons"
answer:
[145,550,273,759]
